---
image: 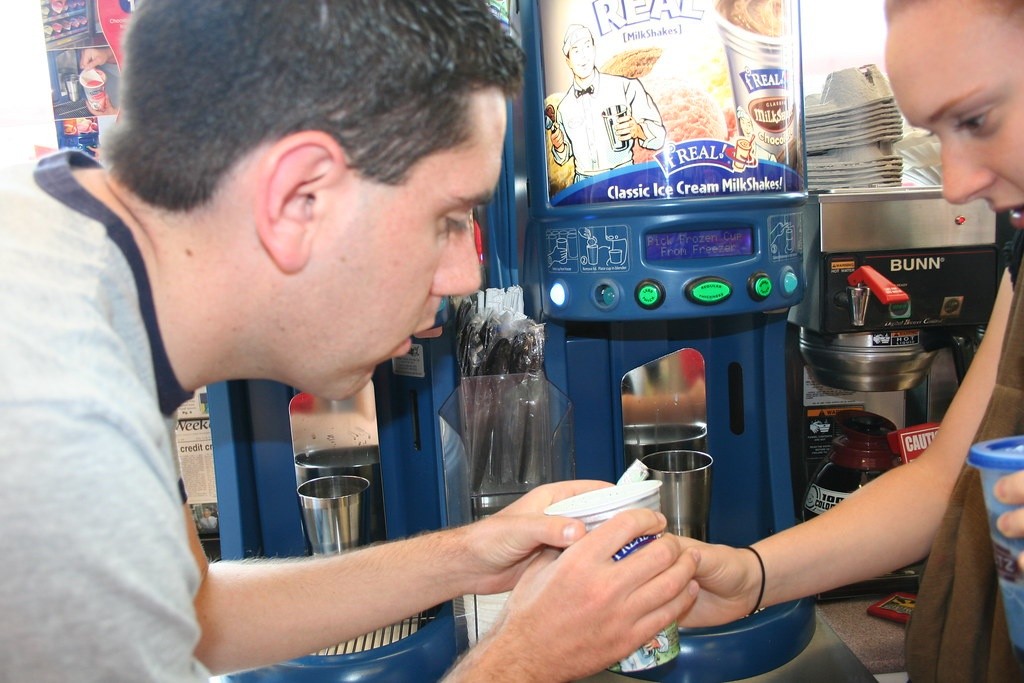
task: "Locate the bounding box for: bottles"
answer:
[802,409,895,523]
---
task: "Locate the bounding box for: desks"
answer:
[189,539,924,683]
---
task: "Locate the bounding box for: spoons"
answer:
[458,319,543,491]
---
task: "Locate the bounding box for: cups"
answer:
[711,0,798,173]
[641,449,715,543]
[295,444,386,547]
[542,481,681,673]
[965,435,1024,673]
[457,372,551,521]
[296,475,371,554]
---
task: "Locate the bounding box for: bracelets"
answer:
[734,544,766,618]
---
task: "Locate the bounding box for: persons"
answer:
[0,0,701,683]
[622,375,706,427]
[650,1,1023,683]
[78,46,120,115]
